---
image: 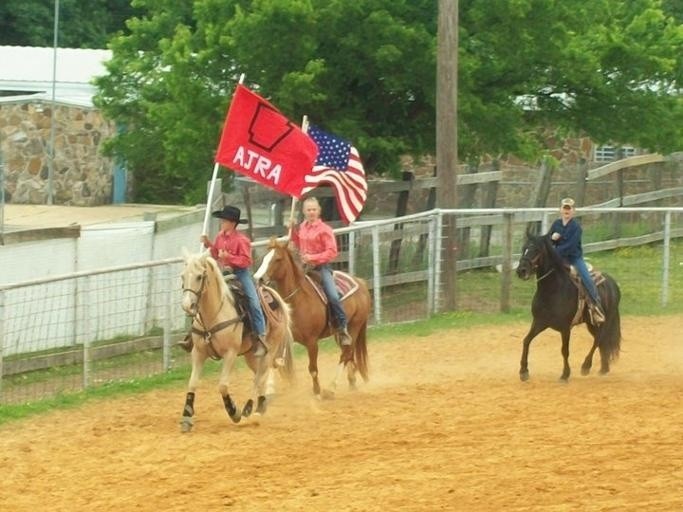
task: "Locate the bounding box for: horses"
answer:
[179,245,296,435]
[252,236,372,403]
[516,231,622,383]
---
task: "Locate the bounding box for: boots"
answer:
[252,334,273,358]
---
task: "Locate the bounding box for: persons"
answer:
[286,196,352,346]
[179,205,268,357]
[545,197,607,326]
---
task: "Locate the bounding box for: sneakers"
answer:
[337,326,354,347]
[177,332,193,353]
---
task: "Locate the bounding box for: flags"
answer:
[215,83,319,199]
[293,115,368,226]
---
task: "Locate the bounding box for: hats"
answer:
[211,205,249,226]
[561,196,576,210]
